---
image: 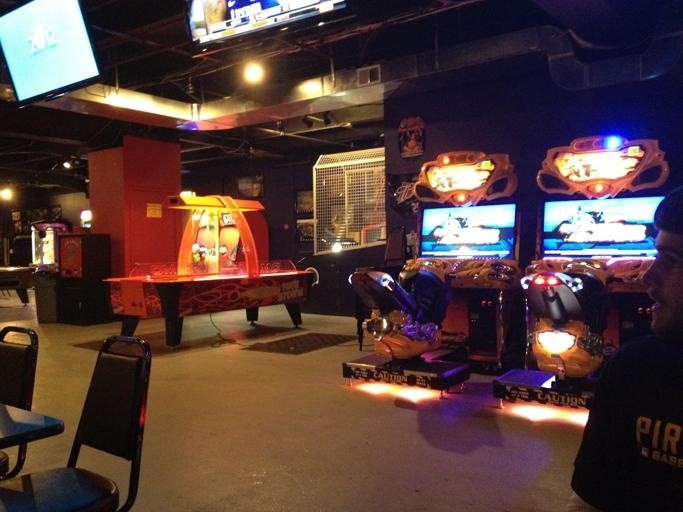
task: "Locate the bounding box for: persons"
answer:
[562,185,682,510]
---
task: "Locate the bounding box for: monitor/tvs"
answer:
[542,194,668,262]
[417,204,516,260]
[181,0,359,59]
[0,0,107,106]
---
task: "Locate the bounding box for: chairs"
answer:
[0,326,149,510]
